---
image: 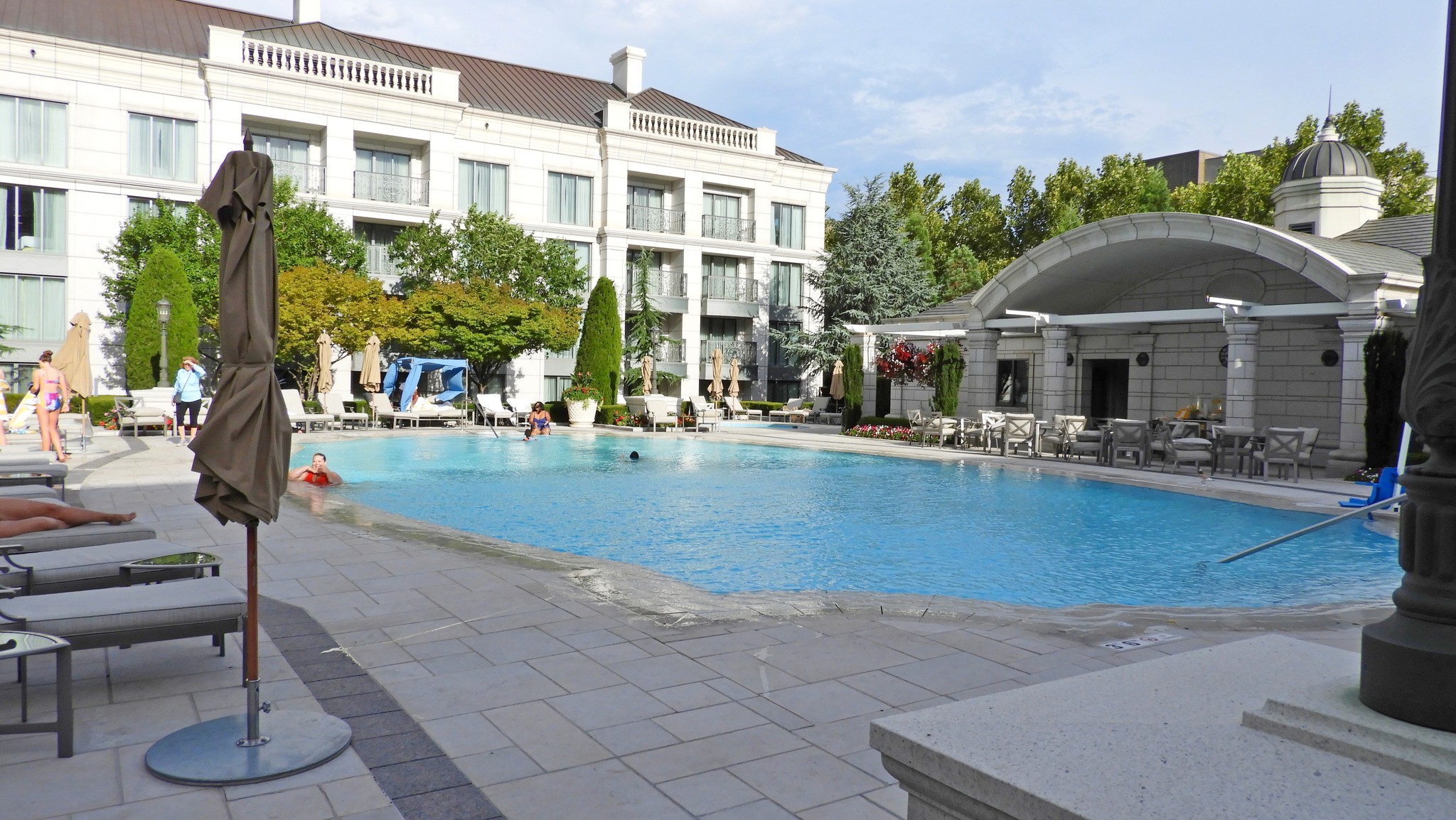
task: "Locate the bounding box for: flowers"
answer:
[561,372,605,412]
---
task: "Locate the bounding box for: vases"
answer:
[564,396,598,428]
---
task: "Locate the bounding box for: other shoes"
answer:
[176,439,186,446]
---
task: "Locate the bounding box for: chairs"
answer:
[282,388,534,432]
[769,397,845,425]
[907,408,1319,484]
[646,396,762,432]
[1338,467,1405,522]
[0,397,247,760]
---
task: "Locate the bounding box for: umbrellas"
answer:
[359,335,381,428]
[707,348,724,432]
[641,356,652,396]
[728,359,739,420]
[316,333,333,415]
[830,360,845,413]
[186,151,292,680]
[50,312,92,452]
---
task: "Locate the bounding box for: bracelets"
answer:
[63,402,68,403]
[533,422,534,424]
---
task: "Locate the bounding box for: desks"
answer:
[942,416,976,449]
[1032,422,1064,458]
[1098,425,1113,466]
[1215,430,1266,479]
[119,551,225,656]
[0,630,74,757]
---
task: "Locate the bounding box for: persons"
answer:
[0,368,8,446]
[287,453,344,488]
[529,401,551,435]
[398,381,418,411]
[620,451,639,462]
[171,357,206,447]
[30,350,69,463]
[523,429,536,441]
[0,498,138,538]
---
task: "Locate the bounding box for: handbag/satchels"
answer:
[173,392,182,403]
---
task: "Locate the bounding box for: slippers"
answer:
[57,456,67,462]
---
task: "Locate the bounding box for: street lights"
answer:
[649,323,660,394]
[156,293,171,388]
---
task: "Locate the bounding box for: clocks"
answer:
[1218,345,1228,368]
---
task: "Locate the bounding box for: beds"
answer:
[400,393,467,429]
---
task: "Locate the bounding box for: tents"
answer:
[383,357,469,413]
